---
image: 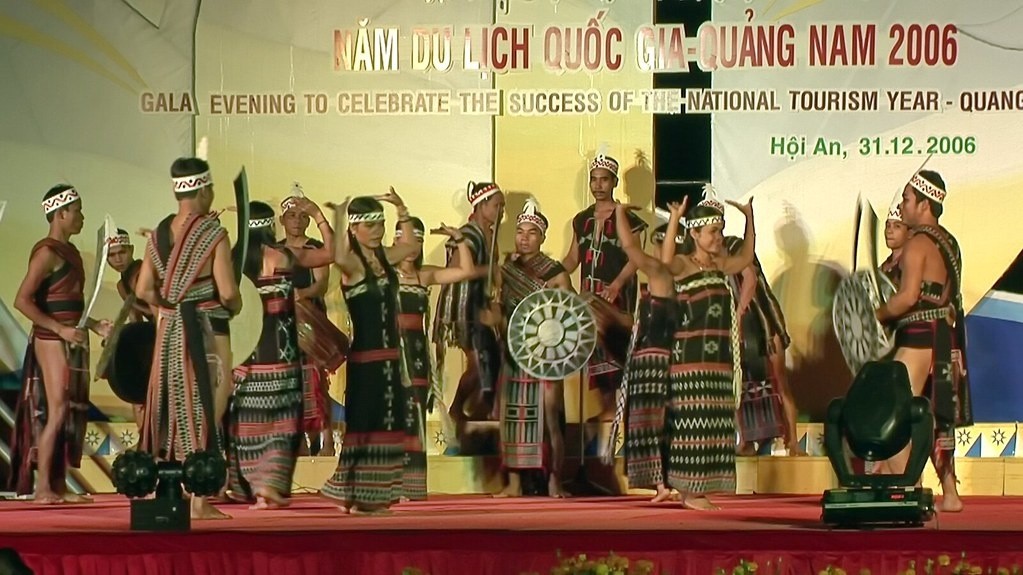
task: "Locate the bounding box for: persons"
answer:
[872,169,968,513]
[105,228,159,430]
[135,156,242,521]
[12,184,112,505]
[208,156,809,516]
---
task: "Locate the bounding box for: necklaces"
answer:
[397,264,417,278]
[690,252,714,272]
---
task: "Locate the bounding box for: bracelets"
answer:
[317,219,326,228]
[398,206,411,222]
[455,236,465,244]
